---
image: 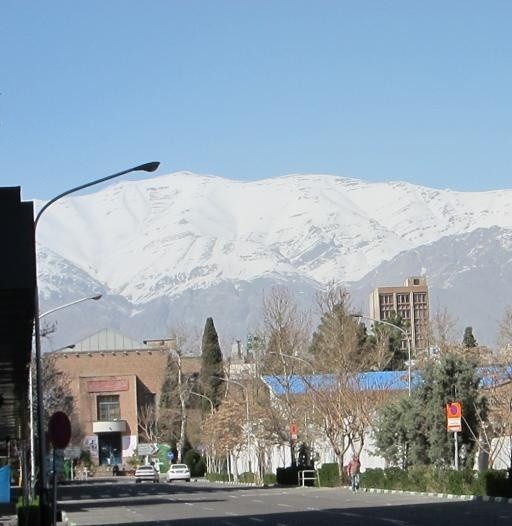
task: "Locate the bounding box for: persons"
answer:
[347,454,361,493]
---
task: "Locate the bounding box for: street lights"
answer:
[30,294,101,502]
[34,161,159,526]
[150,406,185,462]
[269,351,316,467]
[184,390,213,473]
[42,369,63,387]
[207,375,251,473]
[349,313,411,471]
[44,343,75,490]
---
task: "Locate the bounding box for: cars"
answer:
[132,465,160,484]
[166,464,191,482]
